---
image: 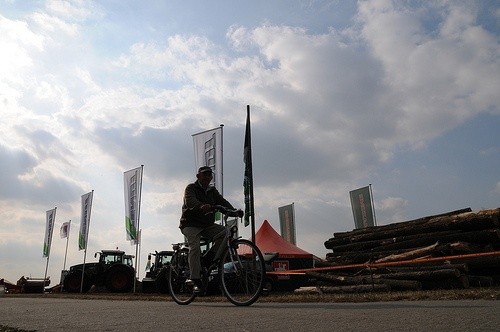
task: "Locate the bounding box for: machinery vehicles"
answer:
[63,250,135,293]
[142,250,182,296]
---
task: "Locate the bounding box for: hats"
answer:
[199,166,212,173]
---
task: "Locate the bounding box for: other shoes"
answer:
[192,284,202,292]
[201,255,217,270]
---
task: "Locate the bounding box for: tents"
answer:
[234,219,326,292]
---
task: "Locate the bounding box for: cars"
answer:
[205,260,279,296]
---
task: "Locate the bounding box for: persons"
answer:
[178,166,244,295]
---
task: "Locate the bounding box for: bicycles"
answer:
[166,204,266,307]
